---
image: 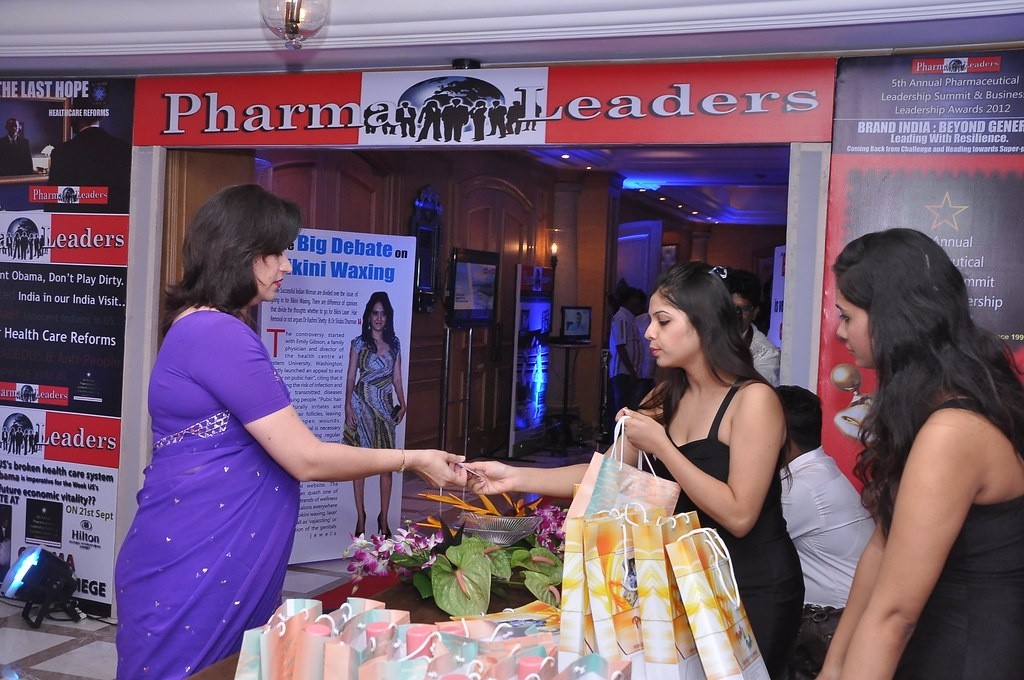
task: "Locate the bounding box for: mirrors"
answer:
[410,185,443,314]
[0,95,72,183]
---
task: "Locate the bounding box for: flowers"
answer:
[529,505,569,555]
[343,521,561,617]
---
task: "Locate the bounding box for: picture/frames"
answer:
[561,306,592,341]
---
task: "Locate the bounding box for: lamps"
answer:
[550,243,558,304]
[257,0,331,51]
[1,546,82,628]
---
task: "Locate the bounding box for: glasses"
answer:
[735,304,758,313]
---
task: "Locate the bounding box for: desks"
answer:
[548,344,597,457]
[180,542,562,679]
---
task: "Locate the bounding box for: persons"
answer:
[773,386,875,609]
[813,227,1024,680]
[342,292,405,537]
[0,118,34,176]
[2,427,38,455]
[569,313,585,331]
[608,288,656,444]
[461,262,806,680]
[48,95,129,214]
[116,185,468,679]
[723,270,779,384]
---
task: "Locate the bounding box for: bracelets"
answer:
[397,448,405,472]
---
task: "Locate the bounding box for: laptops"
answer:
[561,305,592,344]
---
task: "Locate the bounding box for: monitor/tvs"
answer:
[447,247,500,328]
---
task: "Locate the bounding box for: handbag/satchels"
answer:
[791,603,845,675]
[556,500,770,680]
[233,597,632,680]
[561,413,682,533]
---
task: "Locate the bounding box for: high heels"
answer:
[377,513,391,540]
[355,512,366,538]
